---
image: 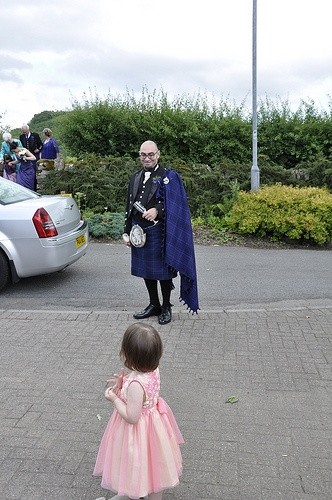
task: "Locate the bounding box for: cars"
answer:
[0,175,89,290]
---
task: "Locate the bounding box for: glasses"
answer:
[140,150,158,159]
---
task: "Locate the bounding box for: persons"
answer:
[18,125,44,161]
[1,154,17,183]
[9,141,37,191]
[0,132,22,173]
[92,321,187,500]
[122,140,183,326]
[40,127,59,160]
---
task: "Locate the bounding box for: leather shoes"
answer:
[158,306,172,325]
[133,304,162,319]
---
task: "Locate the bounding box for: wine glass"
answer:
[133,201,159,226]
